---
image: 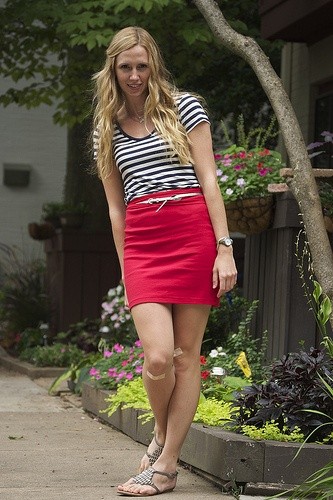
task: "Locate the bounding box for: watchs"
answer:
[216,238,233,249]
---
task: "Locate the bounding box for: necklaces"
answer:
[130,113,144,122]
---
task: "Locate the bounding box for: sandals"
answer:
[137,434,165,473]
[117,467,178,496]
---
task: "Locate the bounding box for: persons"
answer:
[91,26,238,497]
